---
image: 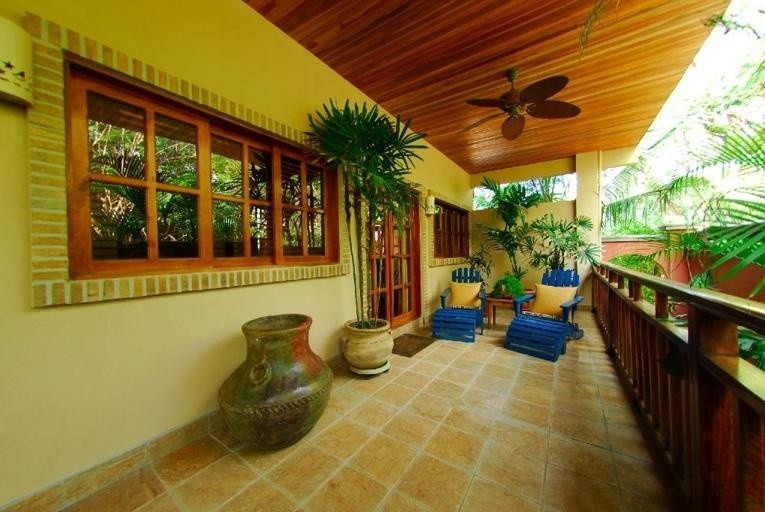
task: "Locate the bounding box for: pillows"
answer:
[530,282,579,321]
[445,281,483,310]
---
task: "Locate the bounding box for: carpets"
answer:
[389,333,435,358]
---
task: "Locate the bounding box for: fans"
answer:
[455,66,584,143]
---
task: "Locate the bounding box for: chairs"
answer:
[502,269,586,363]
[430,266,488,343]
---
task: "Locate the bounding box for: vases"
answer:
[216,309,337,455]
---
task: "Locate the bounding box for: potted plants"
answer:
[302,98,430,378]
[474,171,563,311]
[492,276,525,300]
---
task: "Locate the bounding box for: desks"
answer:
[485,289,531,330]
